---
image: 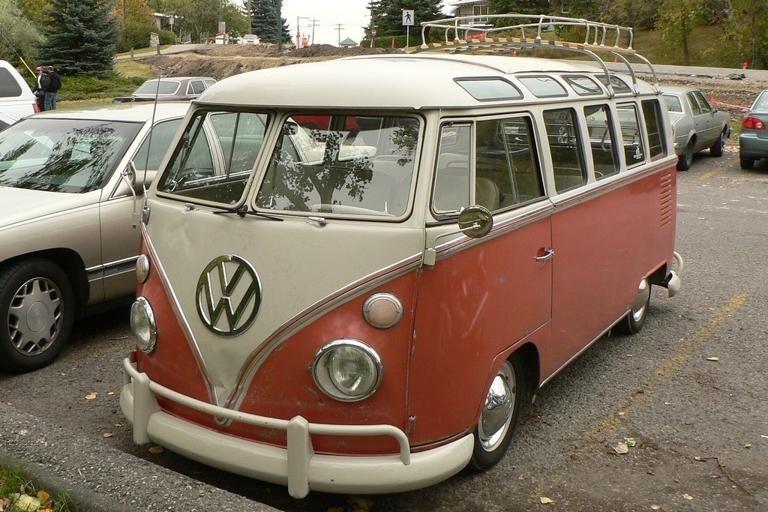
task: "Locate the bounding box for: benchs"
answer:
[367,149,614,218]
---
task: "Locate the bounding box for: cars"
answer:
[130,75,218,100]
[0,59,41,130]
[241,34,262,44]
[0,101,378,370]
[585,87,768,172]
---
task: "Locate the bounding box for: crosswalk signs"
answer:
[402,10,415,25]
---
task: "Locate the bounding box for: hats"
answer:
[36,66,44,71]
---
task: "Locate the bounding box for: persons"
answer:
[31,66,50,112]
[44,65,62,111]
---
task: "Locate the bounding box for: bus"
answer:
[120,15,685,497]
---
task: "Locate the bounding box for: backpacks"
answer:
[48,74,62,91]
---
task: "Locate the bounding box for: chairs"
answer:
[89,141,122,164]
